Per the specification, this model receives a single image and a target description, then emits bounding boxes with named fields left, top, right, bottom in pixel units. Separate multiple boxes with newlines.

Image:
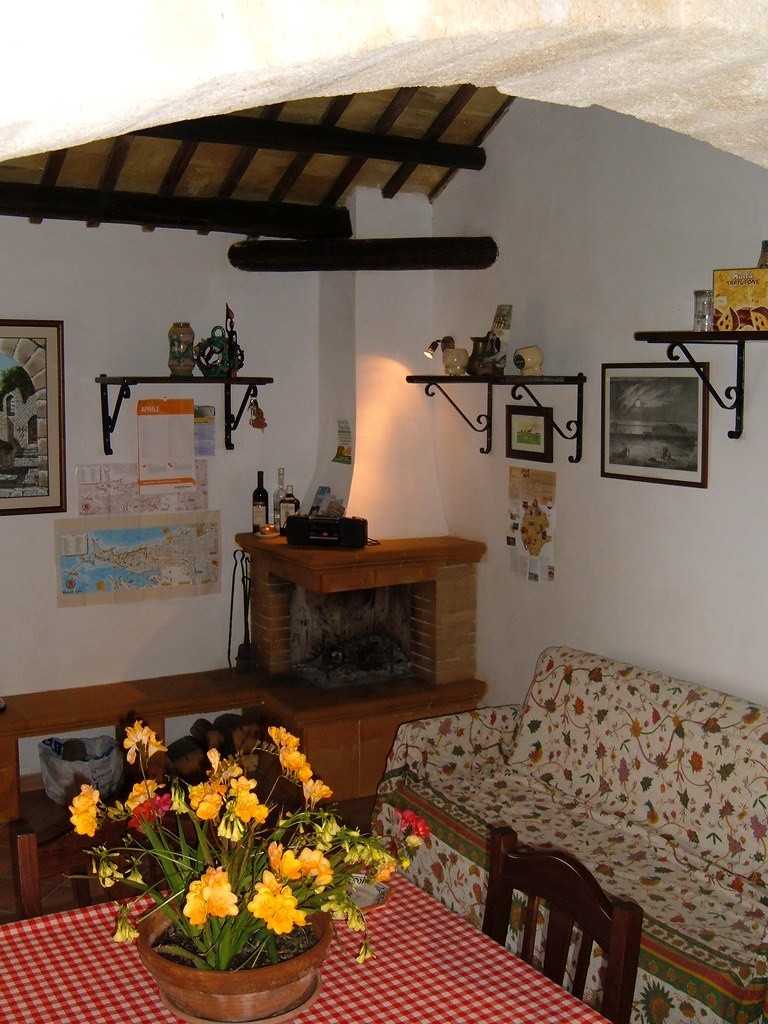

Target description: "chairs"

left=478, top=825, right=644, bottom=1024
left=8, top=801, right=223, bottom=918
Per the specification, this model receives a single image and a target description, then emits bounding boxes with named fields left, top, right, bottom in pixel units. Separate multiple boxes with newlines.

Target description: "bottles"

left=273, top=468, right=287, bottom=532
left=467, top=336, right=487, bottom=376
left=279, top=485, right=300, bottom=536
left=252, top=470, right=268, bottom=532
left=168, top=321, right=195, bottom=373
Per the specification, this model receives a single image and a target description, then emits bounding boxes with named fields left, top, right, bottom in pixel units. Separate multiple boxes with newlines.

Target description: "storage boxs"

left=713, top=268, right=768, bottom=331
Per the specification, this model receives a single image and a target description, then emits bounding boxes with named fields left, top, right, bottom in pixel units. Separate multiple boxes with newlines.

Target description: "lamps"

left=424, top=336, right=455, bottom=359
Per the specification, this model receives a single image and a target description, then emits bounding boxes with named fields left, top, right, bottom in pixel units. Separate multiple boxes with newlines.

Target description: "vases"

left=134, top=900, right=333, bottom=1024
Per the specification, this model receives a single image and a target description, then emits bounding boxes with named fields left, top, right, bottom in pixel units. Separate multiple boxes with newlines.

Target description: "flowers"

left=64, top=716, right=430, bottom=970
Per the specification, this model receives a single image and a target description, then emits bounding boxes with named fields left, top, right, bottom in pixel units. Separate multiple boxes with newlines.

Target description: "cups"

left=693, top=290, right=714, bottom=332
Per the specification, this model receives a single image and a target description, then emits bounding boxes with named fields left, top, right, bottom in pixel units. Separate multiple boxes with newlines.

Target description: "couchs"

left=366, top=644, right=768, bottom=1024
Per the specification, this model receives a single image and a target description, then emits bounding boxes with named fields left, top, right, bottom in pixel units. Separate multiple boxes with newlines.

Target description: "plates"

left=255, top=532, right=280, bottom=538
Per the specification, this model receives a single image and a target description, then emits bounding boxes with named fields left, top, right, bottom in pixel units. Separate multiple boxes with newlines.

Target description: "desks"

left=0, top=869, right=615, bottom=1024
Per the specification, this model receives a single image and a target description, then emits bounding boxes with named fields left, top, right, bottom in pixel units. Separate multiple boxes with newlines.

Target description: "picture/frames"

left=599, top=362, right=711, bottom=489
left=504, top=403, right=553, bottom=463
left=0, top=318, right=67, bottom=517
